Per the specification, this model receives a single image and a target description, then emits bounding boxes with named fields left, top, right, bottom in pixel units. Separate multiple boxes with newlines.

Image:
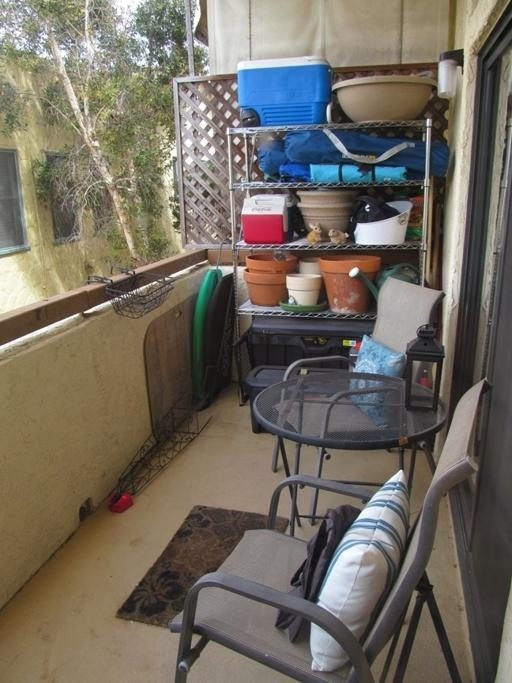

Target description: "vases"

left=244, top=191, right=381, bottom=315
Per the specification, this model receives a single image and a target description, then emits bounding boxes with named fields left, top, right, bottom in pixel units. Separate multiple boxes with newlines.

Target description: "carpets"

left=115, top=505, right=289, bottom=628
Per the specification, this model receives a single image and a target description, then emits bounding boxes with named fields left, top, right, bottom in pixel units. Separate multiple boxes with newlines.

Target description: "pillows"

left=309, top=469, right=410, bottom=672
left=349, top=335, right=405, bottom=426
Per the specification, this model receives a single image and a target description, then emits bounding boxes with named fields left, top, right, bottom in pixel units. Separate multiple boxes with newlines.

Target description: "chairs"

left=169, top=378, right=494, bottom=683
left=272, top=276, right=446, bottom=472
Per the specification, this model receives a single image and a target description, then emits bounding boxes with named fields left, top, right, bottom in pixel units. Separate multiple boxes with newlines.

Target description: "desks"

left=252, top=372, right=450, bottom=536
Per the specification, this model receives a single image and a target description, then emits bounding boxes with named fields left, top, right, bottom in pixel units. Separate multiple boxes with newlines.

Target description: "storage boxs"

left=237, top=56, right=332, bottom=127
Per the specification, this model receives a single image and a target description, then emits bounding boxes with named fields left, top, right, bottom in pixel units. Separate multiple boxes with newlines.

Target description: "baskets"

left=104, top=271, right=176, bottom=319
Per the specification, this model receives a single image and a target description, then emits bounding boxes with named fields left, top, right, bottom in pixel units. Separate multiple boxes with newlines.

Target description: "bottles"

left=437, top=52, right=457, bottom=99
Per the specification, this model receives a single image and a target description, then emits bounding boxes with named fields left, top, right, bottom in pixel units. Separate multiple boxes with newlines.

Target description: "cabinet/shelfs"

left=226, top=118, right=432, bottom=405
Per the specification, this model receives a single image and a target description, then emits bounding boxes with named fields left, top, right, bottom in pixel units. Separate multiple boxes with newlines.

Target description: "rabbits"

left=307, top=224, right=348, bottom=242
